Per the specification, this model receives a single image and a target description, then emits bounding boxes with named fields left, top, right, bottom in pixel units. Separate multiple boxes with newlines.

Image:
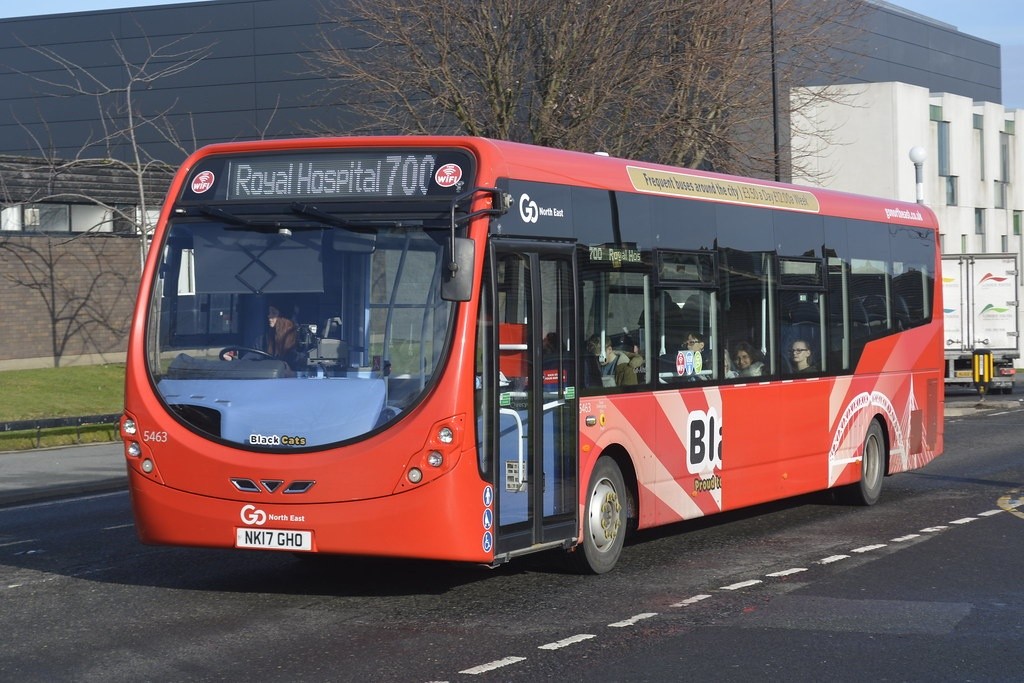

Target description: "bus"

left=119, top=136, right=944, bottom=574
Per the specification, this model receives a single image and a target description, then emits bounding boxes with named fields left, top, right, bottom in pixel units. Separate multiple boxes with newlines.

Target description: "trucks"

left=935, top=252, right=1020, bottom=395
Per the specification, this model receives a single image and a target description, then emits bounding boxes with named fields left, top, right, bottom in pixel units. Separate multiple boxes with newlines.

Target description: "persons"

left=542, top=292, right=817, bottom=389
left=222, top=305, right=307, bottom=372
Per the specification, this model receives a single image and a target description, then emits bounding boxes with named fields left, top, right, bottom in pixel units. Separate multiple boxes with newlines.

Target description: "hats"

left=264, top=296, right=296, bottom=315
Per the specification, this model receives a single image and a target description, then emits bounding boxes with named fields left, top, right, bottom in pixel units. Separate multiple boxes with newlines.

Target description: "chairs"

left=546, top=289, right=909, bottom=390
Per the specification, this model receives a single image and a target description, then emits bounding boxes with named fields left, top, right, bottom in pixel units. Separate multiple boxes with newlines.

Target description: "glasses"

left=790, top=348, right=808, bottom=354
left=682, top=339, right=702, bottom=348
